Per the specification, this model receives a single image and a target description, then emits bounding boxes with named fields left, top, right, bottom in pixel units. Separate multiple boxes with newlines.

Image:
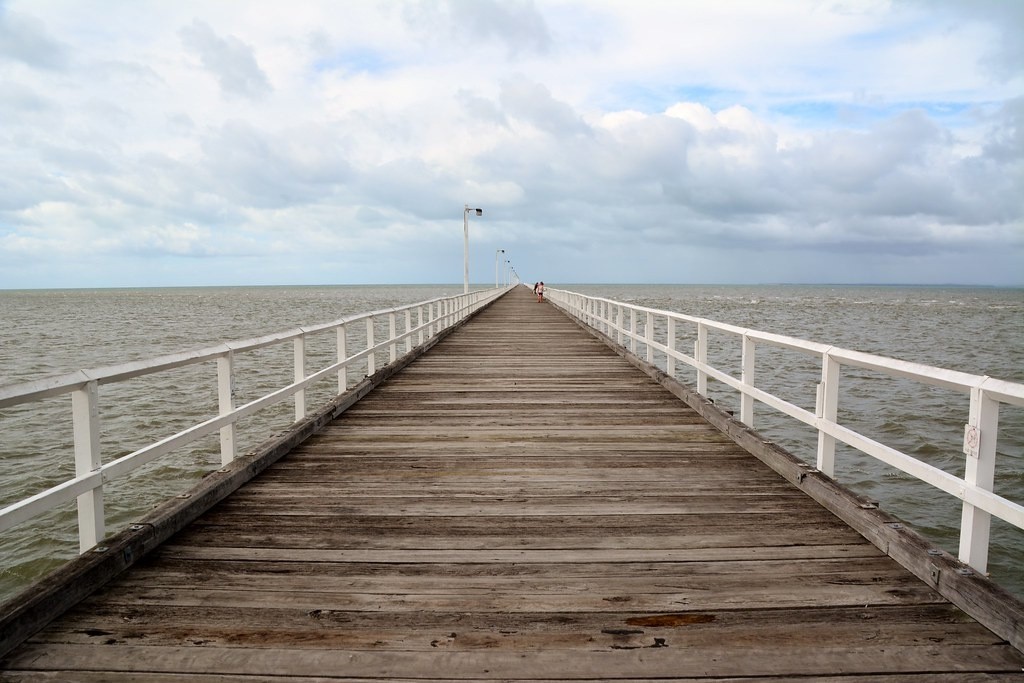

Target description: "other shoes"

left=541, top=301, right=544, bottom=303
left=538, top=301, right=540, bottom=303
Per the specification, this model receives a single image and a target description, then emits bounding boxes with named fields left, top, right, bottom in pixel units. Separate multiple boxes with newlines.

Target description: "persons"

left=535, top=281, right=544, bottom=303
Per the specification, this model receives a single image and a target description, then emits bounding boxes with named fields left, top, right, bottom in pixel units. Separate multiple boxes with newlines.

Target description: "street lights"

left=463, top=207, right=483, bottom=294
left=504, top=261, right=520, bottom=287
left=495, top=249, right=505, bottom=288
